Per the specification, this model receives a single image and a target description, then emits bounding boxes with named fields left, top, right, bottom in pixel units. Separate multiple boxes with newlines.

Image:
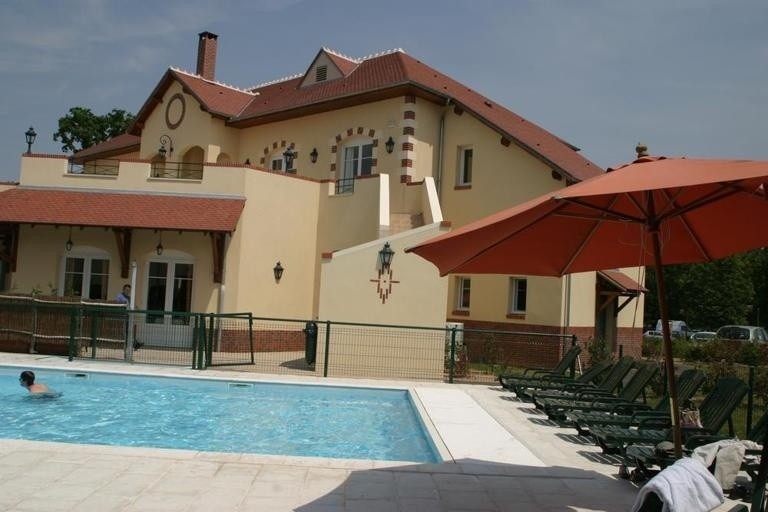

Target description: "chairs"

left=499, top=344, right=768, bottom=512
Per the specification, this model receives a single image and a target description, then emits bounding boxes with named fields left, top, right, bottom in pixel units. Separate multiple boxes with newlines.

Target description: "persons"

left=117, top=285, right=144, bottom=351
left=19, top=371, right=58, bottom=398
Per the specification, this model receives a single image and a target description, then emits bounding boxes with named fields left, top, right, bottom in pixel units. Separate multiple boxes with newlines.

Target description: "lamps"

left=385, top=137, right=395, bottom=153
left=156, top=229, right=163, bottom=255
left=274, top=261, right=284, bottom=279
left=158, top=135, right=173, bottom=160
left=378, top=242, right=396, bottom=265
left=283, top=146, right=296, bottom=170
left=66, top=226, right=73, bottom=250
left=244, top=159, right=251, bottom=165
left=25, top=125, right=37, bottom=152
left=310, top=148, right=318, bottom=163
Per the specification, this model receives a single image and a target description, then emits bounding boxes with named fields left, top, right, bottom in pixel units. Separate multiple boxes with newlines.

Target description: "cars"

left=643, top=319, right=768, bottom=344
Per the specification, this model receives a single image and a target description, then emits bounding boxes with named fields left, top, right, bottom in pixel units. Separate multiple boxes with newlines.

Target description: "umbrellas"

left=403, top=140, right=765, bottom=458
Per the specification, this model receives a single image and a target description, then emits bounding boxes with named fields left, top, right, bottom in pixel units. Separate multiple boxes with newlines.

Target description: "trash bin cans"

left=305, top=321, right=318, bottom=365
